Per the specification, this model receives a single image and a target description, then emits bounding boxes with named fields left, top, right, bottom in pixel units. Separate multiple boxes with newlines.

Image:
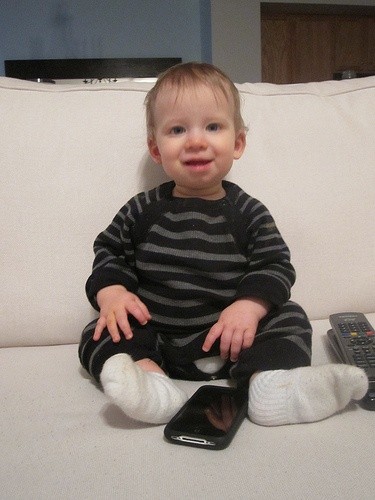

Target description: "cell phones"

left=163, top=385, right=248, bottom=450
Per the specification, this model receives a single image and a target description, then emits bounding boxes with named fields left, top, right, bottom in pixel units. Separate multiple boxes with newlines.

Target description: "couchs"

left=0, top=75, right=375, bottom=500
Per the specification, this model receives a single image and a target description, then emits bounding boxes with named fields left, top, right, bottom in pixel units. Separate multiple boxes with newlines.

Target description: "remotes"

left=327, top=329, right=375, bottom=411
left=330, top=311, right=375, bottom=389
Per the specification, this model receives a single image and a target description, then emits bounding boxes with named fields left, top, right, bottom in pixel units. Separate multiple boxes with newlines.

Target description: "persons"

left=206, top=393, right=238, bottom=432
left=77, top=61, right=367, bottom=427
